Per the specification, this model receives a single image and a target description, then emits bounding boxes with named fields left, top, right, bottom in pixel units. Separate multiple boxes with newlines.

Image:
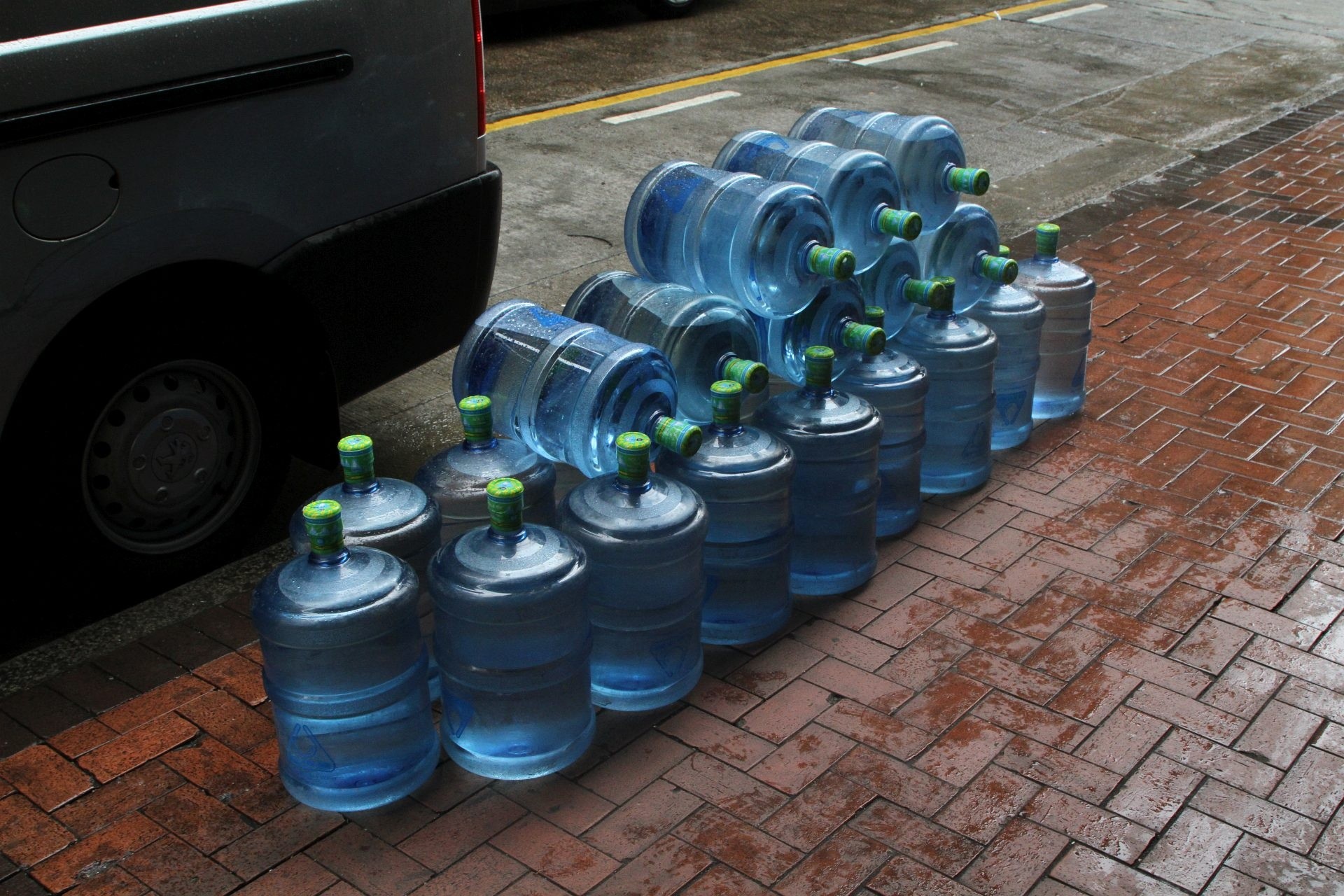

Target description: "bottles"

left=408, top=394, right=556, bottom=572
left=250, top=500, right=442, bottom=811
left=1004, top=222, right=1096, bottom=418
left=287, top=433, right=446, bottom=705
left=451, top=104, right=1047, bottom=712
left=424, top=476, right=599, bottom=782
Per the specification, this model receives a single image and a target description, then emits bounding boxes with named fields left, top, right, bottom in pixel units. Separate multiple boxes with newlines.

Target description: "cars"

left=1, top=1, right=508, bottom=611
